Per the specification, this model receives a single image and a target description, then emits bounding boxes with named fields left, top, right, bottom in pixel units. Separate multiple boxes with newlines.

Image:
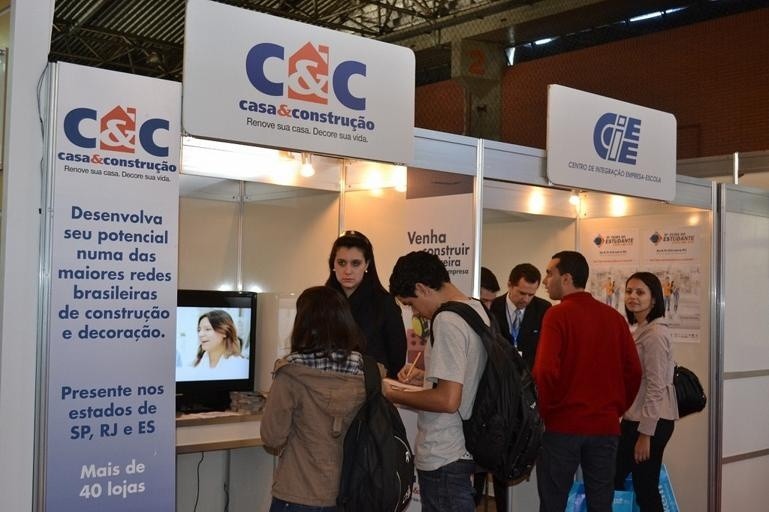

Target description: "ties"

left=511, top=309, right=521, bottom=346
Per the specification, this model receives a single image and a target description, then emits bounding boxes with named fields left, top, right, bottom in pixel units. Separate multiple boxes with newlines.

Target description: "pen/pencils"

left=406, top=351, right=422, bottom=377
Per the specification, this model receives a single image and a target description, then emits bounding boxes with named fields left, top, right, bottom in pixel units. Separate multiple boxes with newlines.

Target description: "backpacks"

left=430, top=296, right=543, bottom=483
left=336, top=353, right=415, bottom=512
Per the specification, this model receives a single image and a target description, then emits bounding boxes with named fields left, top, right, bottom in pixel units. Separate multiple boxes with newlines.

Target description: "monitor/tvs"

left=176, top=289, right=257, bottom=414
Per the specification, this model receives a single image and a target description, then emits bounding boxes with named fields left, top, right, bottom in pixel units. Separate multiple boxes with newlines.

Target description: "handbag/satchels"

left=673, top=365, right=707, bottom=418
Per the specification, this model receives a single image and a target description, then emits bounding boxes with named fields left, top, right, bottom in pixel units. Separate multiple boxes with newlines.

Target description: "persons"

left=531, top=250, right=644, bottom=512
left=602, top=277, right=620, bottom=312
left=661, top=277, right=680, bottom=311
left=382, top=250, right=497, bottom=511
left=615, top=272, right=680, bottom=512
left=185, top=307, right=251, bottom=380
left=325, top=230, right=408, bottom=379
left=481, top=264, right=499, bottom=311
left=260, top=285, right=382, bottom=511
left=493, top=263, right=551, bottom=368
